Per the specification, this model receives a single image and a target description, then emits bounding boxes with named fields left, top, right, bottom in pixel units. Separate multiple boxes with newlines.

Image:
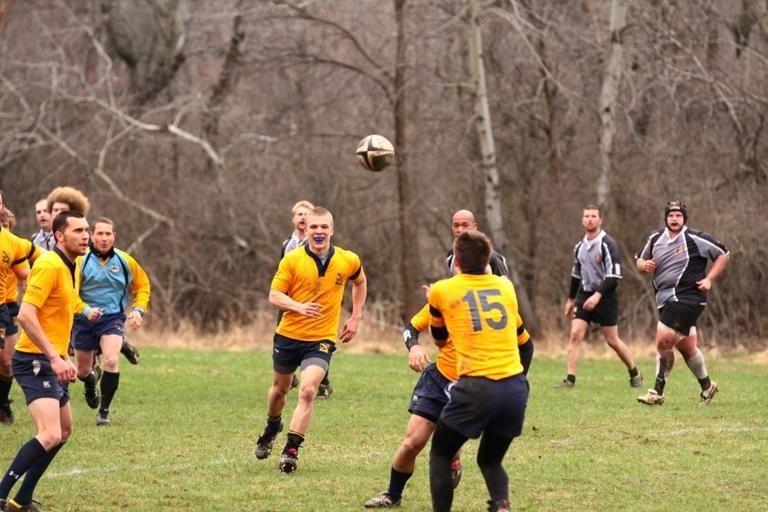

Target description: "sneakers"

left=254, top=376, right=334, bottom=460
left=120, top=336, right=141, bottom=367
left=362, top=451, right=513, bottom=512
left=94, top=406, right=112, bottom=427
left=551, top=377, right=577, bottom=389
left=629, top=371, right=646, bottom=389
left=694, top=381, right=720, bottom=407
left=637, top=387, right=666, bottom=407
left=278, top=446, right=299, bottom=472
left=82, top=370, right=101, bottom=409
left=0, top=497, right=42, bottom=512
left=0, top=395, right=16, bottom=426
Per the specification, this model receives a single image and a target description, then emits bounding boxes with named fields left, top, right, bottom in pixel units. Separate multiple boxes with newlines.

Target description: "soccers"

left=357, top=136, right=394, bottom=171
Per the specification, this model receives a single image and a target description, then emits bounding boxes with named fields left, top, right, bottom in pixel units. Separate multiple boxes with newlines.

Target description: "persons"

left=276, top=200, right=333, bottom=398
left=0, top=187, right=150, bottom=429
left=559, top=202, right=643, bottom=389
left=253, top=207, right=367, bottom=474
left=363, top=285, right=536, bottom=505
left=447, top=209, right=510, bottom=280
left=427, top=231, right=529, bottom=510
left=634, top=200, right=731, bottom=404
left=1, top=209, right=89, bottom=511
